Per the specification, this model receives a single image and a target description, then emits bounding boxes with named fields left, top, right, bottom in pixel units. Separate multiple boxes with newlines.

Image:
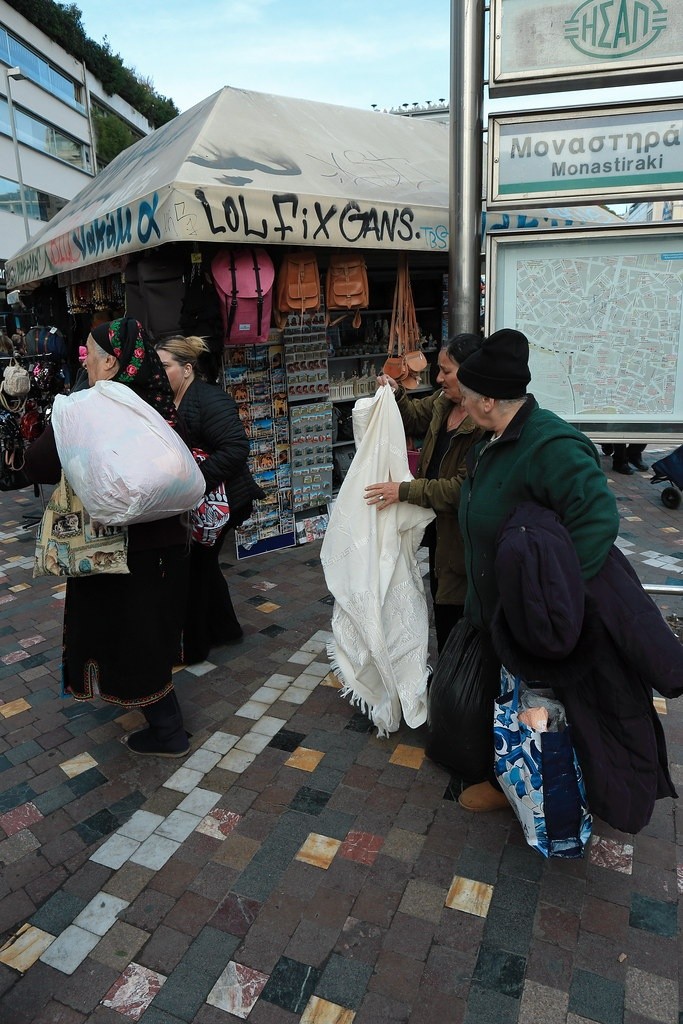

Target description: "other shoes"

left=602, top=443, right=614, bottom=456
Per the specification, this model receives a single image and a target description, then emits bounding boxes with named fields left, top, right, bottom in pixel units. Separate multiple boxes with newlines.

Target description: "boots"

left=125, top=687, right=191, bottom=758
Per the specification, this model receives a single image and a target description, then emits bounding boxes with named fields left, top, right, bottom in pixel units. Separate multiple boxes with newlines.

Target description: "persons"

left=280, top=450, right=288, bottom=465
left=154, top=335, right=265, bottom=666
left=25, top=317, right=190, bottom=756
left=611, top=443, right=650, bottom=474
left=0, top=329, right=43, bottom=489
left=457, top=329, right=619, bottom=812
left=365, top=333, right=488, bottom=731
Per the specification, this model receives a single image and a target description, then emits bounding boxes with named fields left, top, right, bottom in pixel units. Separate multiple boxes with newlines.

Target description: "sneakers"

left=457, top=780, right=511, bottom=812
left=626, top=447, right=650, bottom=470
left=612, top=464, right=634, bottom=475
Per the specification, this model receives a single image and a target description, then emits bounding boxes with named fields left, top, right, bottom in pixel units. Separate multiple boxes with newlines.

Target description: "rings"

left=380, top=495, right=384, bottom=501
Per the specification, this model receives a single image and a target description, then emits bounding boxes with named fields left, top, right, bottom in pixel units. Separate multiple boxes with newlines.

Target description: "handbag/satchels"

left=52, top=379, right=208, bottom=526
left=0, top=281, right=78, bottom=498
left=383, top=350, right=427, bottom=389
left=181, top=447, right=230, bottom=547
left=493, top=664, right=592, bottom=860
left=32, top=468, right=130, bottom=579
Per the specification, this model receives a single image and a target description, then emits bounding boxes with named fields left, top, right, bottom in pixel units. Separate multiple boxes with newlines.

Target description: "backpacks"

left=124, top=244, right=370, bottom=386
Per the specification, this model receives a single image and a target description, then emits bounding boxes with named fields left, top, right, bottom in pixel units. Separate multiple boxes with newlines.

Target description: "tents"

left=4, top=86, right=627, bottom=289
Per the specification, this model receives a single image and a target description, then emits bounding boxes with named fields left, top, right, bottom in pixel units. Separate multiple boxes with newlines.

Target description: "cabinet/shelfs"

left=222, top=304, right=431, bottom=560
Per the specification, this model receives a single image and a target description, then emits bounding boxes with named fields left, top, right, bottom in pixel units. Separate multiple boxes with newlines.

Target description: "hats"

left=456, top=328, right=531, bottom=399
